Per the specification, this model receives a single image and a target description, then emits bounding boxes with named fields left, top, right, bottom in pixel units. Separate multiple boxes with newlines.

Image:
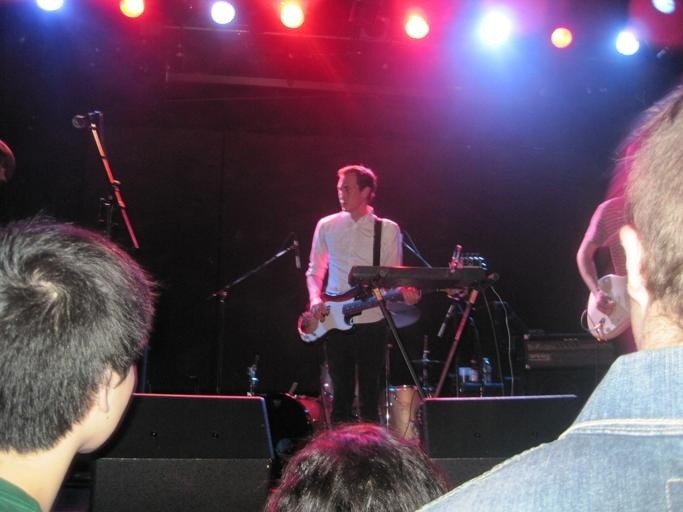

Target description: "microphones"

left=73, top=110, right=103, bottom=128
left=293, top=237, right=302, bottom=270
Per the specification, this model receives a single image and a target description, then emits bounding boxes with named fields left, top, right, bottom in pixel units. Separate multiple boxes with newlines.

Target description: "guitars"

left=296, top=286, right=467, bottom=343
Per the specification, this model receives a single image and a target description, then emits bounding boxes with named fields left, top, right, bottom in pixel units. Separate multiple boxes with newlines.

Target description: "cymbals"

left=402, top=354, right=451, bottom=368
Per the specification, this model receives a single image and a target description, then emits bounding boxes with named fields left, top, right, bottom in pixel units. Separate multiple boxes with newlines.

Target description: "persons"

left=415, top=90, right=683, bottom=512
left=0, top=218, right=153, bottom=511
left=265, top=421, right=447, bottom=511
left=575, top=193, right=638, bottom=354
left=304, top=164, right=424, bottom=429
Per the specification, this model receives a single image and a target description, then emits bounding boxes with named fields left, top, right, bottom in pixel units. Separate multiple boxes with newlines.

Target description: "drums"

left=250, top=392, right=325, bottom=459
left=379, top=385, right=435, bottom=447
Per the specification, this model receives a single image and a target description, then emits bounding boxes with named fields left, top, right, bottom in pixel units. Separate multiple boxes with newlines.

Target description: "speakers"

left=90, top=393, right=276, bottom=511
left=419, top=395, right=581, bottom=501
